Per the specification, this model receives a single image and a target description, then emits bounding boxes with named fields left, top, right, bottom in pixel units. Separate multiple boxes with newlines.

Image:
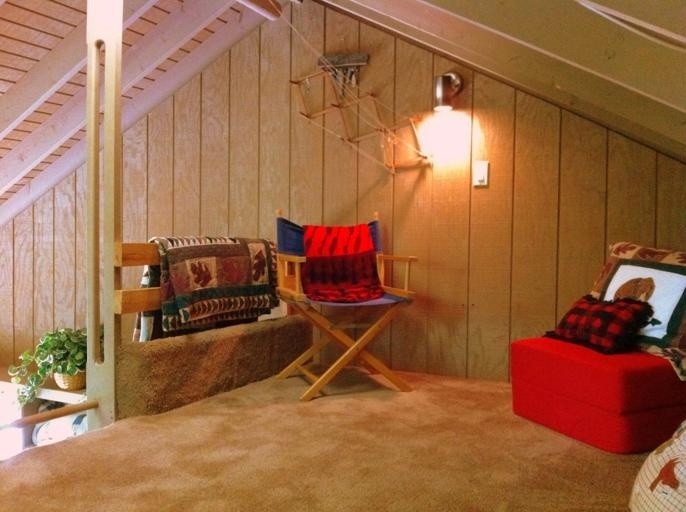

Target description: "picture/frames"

left=550, top=297, right=651, bottom=353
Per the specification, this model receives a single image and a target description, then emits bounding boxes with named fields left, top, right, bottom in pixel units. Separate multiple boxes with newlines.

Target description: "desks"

left=15, top=373, right=87, bottom=450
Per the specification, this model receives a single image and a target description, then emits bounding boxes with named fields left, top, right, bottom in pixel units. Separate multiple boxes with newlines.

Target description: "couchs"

left=512, top=242, right=686, bottom=453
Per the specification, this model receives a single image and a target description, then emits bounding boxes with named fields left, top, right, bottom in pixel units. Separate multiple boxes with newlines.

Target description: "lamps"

left=431, top=72, right=461, bottom=113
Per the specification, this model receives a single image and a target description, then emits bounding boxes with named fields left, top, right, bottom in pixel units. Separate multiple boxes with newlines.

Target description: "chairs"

left=273, top=210, right=416, bottom=401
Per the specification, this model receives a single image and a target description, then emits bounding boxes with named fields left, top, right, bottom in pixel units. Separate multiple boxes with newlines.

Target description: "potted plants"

left=10, top=326, right=104, bottom=399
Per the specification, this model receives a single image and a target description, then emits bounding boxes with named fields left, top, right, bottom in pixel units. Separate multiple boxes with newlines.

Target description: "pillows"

left=589, top=259, right=686, bottom=352
